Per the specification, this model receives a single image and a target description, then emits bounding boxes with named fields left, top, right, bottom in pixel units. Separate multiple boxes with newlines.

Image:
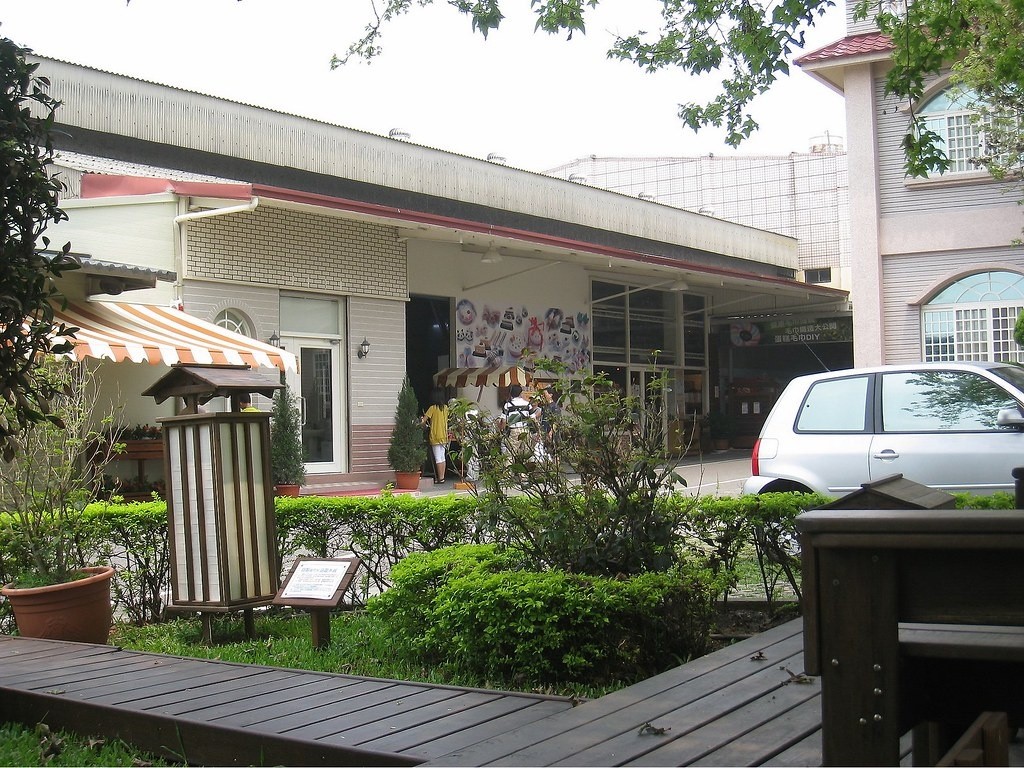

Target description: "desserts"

left=455, top=303, right=528, bottom=368
left=545, top=309, right=591, bottom=374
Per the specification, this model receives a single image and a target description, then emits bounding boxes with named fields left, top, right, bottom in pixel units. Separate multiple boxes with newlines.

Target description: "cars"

left=742, top=362, right=1024, bottom=565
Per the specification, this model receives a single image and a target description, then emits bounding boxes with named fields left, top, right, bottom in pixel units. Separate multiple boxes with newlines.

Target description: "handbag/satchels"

left=527, top=437, right=553, bottom=463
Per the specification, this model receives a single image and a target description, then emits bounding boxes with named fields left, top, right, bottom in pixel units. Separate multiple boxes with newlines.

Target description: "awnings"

left=0, top=301, right=302, bottom=375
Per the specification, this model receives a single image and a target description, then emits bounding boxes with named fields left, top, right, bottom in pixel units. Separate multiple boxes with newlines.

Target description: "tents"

left=432, top=366, right=530, bottom=403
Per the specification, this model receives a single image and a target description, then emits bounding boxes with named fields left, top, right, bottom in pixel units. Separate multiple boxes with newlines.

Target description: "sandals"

left=435, top=478, right=444, bottom=483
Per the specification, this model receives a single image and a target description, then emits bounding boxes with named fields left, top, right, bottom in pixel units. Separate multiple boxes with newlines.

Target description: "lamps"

left=480, top=239, right=503, bottom=264
left=268, top=330, right=280, bottom=347
left=358, top=336, right=371, bottom=359
left=669, top=272, right=689, bottom=291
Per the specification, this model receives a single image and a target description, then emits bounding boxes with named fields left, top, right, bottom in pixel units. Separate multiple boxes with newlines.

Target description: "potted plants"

left=270, top=381, right=308, bottom=498
left=0, top=342, right=131, bottom=644
left=701, top=408, right=736, bottom=450
left=386, top=370, right=429, bottom=491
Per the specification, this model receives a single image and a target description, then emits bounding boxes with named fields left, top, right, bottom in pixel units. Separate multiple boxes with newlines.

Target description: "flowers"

left=94, top=423, right=162, bottom=441
left=103, top=471, right=166, bottom=494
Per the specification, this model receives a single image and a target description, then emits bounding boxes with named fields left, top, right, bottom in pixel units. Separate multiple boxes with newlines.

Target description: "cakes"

left=529, top=325, right=544, bottom=346
left=507, top=335, right=524, bottom=356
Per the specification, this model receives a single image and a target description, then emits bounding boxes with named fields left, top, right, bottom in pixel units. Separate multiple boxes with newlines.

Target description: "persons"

left=417, top=385, right=562, bottom=491
left=176, top=393, right=262, bottom=415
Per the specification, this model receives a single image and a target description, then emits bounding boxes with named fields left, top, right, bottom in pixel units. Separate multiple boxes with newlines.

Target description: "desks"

left=86, top=439, right=163, bottom=496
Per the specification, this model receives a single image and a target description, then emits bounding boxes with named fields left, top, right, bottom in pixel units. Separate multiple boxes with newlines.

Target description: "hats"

left=529, top=395, right=540, bottom=400
